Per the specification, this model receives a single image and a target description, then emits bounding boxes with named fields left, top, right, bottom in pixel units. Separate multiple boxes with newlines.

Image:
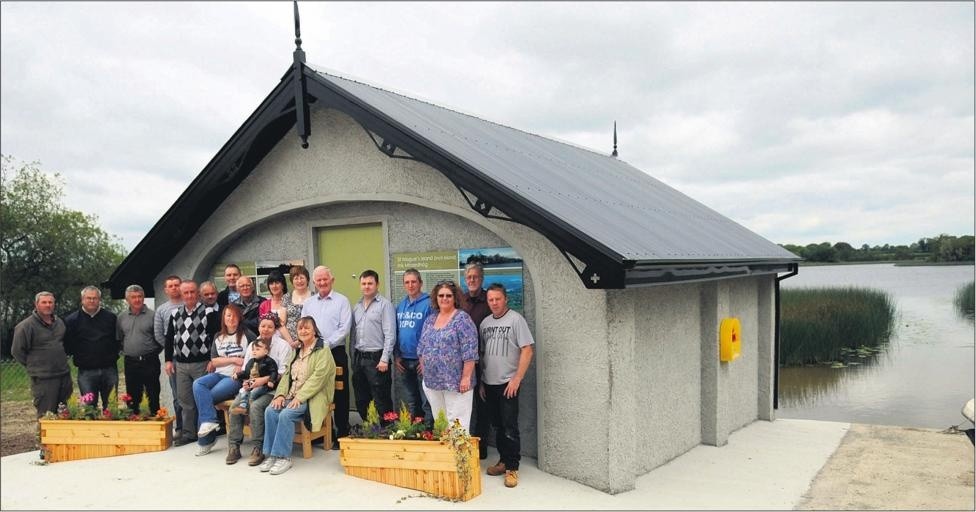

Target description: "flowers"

left=362, top=402, right=449, bottom=441
left=58, top=391, right=168, bottom=421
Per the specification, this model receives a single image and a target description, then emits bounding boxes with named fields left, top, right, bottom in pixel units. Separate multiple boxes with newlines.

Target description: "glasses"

left=437, top=280, right=454, bottom=286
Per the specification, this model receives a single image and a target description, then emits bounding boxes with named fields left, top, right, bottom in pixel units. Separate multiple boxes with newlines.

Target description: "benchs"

left=214, top=366, right=344, bottom=459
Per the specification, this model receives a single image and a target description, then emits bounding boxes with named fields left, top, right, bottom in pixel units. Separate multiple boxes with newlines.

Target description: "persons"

left=9, top=262, right=534, bottom=490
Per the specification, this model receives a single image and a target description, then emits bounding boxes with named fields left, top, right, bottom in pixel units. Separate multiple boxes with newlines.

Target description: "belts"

left=125, top=354, right=159, bottom=361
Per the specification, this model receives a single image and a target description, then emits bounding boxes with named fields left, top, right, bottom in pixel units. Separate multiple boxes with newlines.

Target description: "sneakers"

left=226, top=446, right=242, bottom=465
left=487, top=462, right=506, bottom=476
left=246, top=446, right=264, bottom=466
left=193, top=438, right=219, bottom=457
left=269, top=455, right=293, bottom=475
left=172, top=430, right=198, bottom=446
left=476, top=440, right=488, bottom=460
left=504, top=469, right=519, bottom=488
left=195, top=423, right=221, bottom=437
left=332, top=433, right=348, bottom=451
left=312, top=437, right=324, bottom=446
left=230, top=406, right=249, bottom=416
left=259, top=456, right=278, bottom=472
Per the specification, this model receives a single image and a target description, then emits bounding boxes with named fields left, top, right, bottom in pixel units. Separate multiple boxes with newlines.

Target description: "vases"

left=40, top=418, right=176, bottom=462
left=338, top=437, right=482, bottom=500
